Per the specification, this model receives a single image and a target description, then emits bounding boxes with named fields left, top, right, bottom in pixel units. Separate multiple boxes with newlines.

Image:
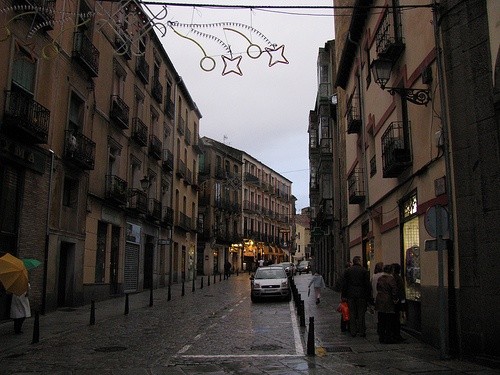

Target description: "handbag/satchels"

left=308, top=287, right=310, bottom=297
left=365, top=305, right=375, bottom=321
left=336, top=310, right=342, bottom=322
left=399, top=311, right=407, bottom=325
left=363, top=282, right=374, bottom=305
left=336, top=291, right=342, bottom=303
left=375, top=293, right=382, bottom=310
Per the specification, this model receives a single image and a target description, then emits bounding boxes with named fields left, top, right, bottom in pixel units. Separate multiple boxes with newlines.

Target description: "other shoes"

left=16, top=331, right=24, bottom=334
left=352, top=335, right=356, bottom=337
left=360, top=335, right=366, bottom=337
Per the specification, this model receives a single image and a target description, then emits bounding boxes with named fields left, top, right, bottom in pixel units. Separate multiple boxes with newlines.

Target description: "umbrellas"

left=0, top=253, right=28, bottom=296
left=22, top=259, right=41, bottom=271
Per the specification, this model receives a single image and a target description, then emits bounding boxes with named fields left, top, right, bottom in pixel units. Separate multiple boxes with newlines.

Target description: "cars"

left=249, top=266, right=291, bottom=302
left=268, top=262, right=296, bottom=281
left=297, top=261, right=311, bottom=274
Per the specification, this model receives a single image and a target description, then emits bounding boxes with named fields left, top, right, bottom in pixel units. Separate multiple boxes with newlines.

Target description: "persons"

left=10, top=283, right=31, bottom=334
left=374, top=264, right=400, bottom=344
left=247, top=258, right=274, bottom=271
left=337, top=297, right=350, bottom=335
left=371, top=262, right=385, bottom=326
left=391, top=263, right=405, bottom=343
left=308, top=270, right=326, bottom=306
left=224, top=259, right=232, bottom=277
left=341, top=256, right=370, bottom=337
